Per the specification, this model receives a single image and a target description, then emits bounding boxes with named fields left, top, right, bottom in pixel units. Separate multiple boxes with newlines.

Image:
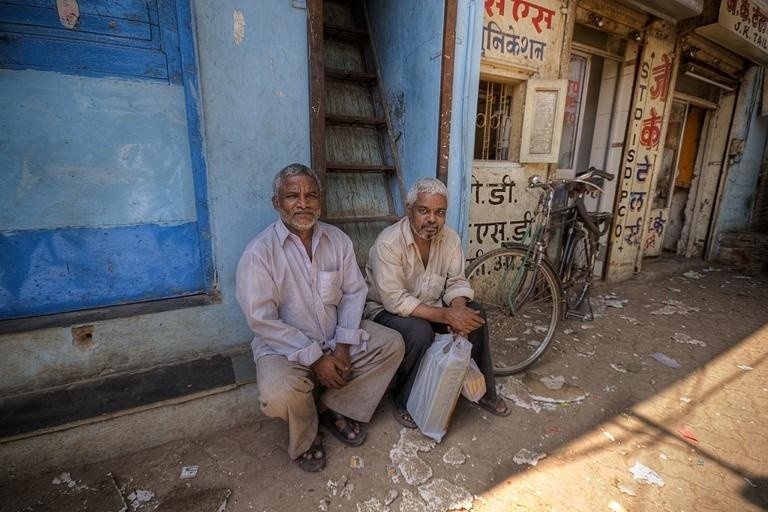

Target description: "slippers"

left=319, top=404, right=367, bottom=447
left=389, top=392, right=418, bottom=430
left=296, top=434, right=326, bottom=472
left=479, top=393, right=512, bottom=418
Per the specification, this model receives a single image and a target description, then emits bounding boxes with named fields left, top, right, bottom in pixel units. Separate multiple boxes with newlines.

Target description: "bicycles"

left=461, top=167, right=615, bottom=376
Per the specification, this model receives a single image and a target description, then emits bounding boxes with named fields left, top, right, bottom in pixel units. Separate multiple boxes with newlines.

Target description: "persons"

left=233, top=162, right=406, bottom=473
left=363, top=176, right=510, bottom=429
